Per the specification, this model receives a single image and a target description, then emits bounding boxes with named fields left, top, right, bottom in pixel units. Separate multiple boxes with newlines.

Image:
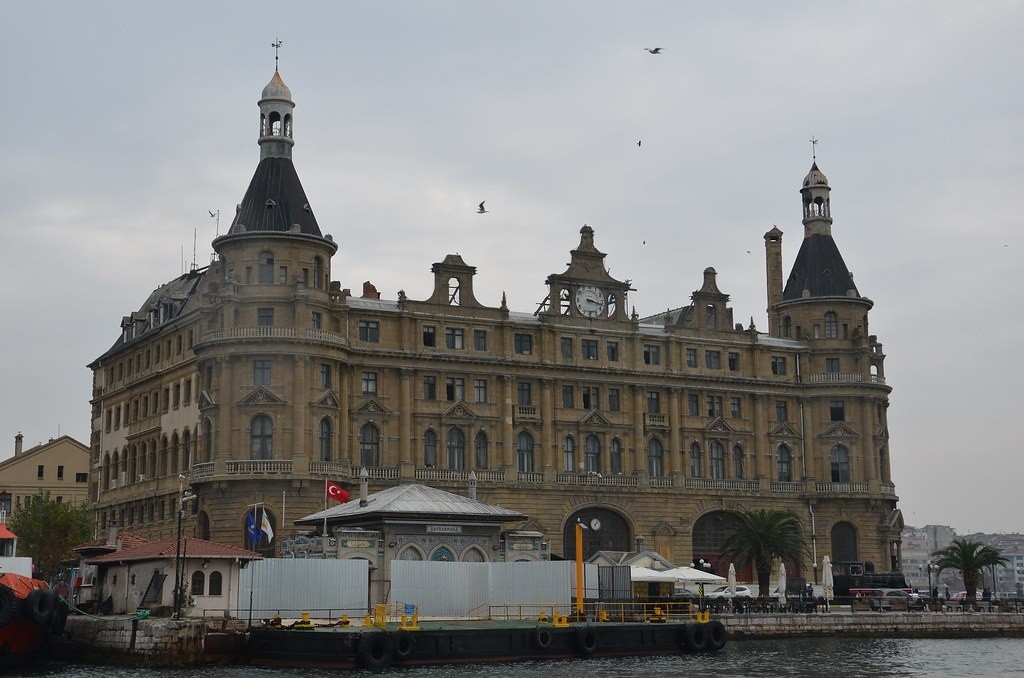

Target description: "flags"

left=246, top=511, right=261, bottom=545
left=327, top=481, right=350, bottom=503
left=261, top=508, right=274, bottom=543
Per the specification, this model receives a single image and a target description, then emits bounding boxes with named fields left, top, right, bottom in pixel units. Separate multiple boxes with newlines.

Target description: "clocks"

left=575, top=285, right=605, bottom=318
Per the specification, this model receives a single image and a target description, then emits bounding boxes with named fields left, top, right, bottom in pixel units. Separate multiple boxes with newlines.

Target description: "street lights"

left=919, top=560, right=939, bottom=597
left=690, top=559, right=712, bottom=597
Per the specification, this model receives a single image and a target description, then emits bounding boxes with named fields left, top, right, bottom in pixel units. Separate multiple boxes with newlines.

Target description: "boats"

left=0, top=510, right=69, bottom=656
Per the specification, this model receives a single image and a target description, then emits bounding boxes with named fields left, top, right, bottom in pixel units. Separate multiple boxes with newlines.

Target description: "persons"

left=982, top=588, right=991, bottom=601
left=945, top=587, right=950, bottom=599
left=633, top=592, right=644, bottom=616
left=933, top=586, right=938, bottom=597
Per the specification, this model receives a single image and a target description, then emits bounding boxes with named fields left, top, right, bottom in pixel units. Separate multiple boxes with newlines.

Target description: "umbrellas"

left=822, top=554, right=835, bottom=613
left=778, top=564, right=786, bottom=606
left=728, top=562, right=737, bottom=612
left=631, top=566, right=727, bottom=610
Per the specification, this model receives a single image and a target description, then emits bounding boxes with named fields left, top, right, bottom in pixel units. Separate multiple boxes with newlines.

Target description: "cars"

left=867, top=589, right=915, bottom=610
left=706, top=586, right=753, bottom=598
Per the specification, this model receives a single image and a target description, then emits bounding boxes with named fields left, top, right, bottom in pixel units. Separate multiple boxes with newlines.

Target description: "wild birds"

left=208, top=209, right=217, bottom=218
left=476, top=201, right=490, bottom=215
left=746, top=250, right=751, bottom=253
left=636, top=140, right=642, bottom=148
left=643, top=47, right=664, bottom=56
left=641, top=241, right=648, bottom=245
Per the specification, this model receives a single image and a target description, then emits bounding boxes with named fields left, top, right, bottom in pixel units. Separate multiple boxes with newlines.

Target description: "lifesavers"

left=33, top=594, right=49, bottom=624
left=0, top=583, right=17, bottom=628
left=684, top=623, right=709, bottom=653
left=392, top=630, right=416, bottom=661
left=358, top=632, right=394, bottom=671
left=705, top=621, right=728, bottom=651
left=578, top=628, right=599, bottom=654
left=52, top=603, right=68, bottom=636
left=533, top=629, right=551, bottom=650
